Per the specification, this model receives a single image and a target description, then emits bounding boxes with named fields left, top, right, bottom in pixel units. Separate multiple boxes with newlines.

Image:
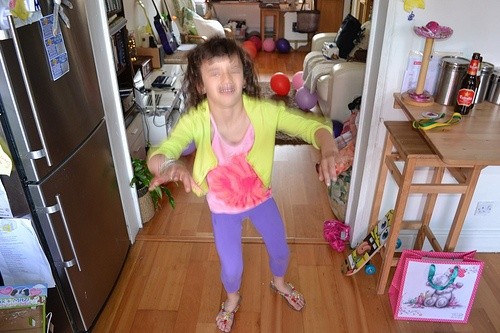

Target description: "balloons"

left=270, top=71, right=317, bottom=110
left=242, top=36, right=291, bottom=60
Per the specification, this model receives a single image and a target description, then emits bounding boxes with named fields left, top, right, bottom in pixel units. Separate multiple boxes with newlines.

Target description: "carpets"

left=258, top=81, right=322, bottom=146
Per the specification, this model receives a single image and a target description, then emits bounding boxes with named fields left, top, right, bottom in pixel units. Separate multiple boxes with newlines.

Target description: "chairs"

left=188, top=26, right=234, bottom=45
left=292, top=10, right=321, bottom=52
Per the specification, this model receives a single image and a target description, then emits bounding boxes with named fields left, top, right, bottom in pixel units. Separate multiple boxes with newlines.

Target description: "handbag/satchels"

left=387, top=249, right=486, bottom=324
left=296, top=0, right=321, bottom=34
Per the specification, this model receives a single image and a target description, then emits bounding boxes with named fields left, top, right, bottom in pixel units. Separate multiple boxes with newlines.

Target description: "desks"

left=259, top=8, right=299, bottom=43
left=134, top=63, right=191, bottom=147
left=393, top=92, right=500, bottom=167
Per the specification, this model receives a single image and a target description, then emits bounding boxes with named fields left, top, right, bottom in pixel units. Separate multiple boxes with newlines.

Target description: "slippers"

left=270, top=279, right=306, bottom=311
left=215, top=295, right=241, bottom=333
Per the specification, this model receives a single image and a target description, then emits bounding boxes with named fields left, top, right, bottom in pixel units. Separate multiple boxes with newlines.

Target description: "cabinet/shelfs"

left=105, top=0, right=141, bottom=130
left=356, top=0, right=372, bottom=25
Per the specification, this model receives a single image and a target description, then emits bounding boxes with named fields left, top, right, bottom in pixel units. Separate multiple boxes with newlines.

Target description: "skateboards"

left=342, top=208, right=401, bottom=276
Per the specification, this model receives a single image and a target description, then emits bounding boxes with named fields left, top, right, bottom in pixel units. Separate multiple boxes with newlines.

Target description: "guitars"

left=136, top=0, right=181, bottom=54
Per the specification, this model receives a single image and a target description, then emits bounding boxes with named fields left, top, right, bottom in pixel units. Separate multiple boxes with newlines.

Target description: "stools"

left=365, top=119, right=482, bottom=295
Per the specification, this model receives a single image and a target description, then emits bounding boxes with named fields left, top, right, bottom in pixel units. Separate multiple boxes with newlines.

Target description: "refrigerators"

left=0, top=9, right=131, bottom=333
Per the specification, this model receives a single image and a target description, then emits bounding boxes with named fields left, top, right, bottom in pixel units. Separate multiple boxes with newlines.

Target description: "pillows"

left=183, top=7, right=204, bottom=34
left=193, top=16, right=227, bottom=45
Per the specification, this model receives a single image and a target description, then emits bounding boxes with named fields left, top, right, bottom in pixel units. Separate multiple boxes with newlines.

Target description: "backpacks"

left=334, top=13, right=367, bottom=59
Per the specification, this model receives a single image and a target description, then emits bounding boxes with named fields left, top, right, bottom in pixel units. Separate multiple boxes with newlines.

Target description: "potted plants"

left=128, top=155, right=176, bottom=224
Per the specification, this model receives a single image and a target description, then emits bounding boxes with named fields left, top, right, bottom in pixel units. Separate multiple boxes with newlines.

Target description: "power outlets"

left=473, top=200, right=494, bottom=216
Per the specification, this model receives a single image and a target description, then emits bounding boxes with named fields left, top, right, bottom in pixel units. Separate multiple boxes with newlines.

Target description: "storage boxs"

left=135, top=44, right=164, bottom=70
left=132, top=56, right=155, bottom=81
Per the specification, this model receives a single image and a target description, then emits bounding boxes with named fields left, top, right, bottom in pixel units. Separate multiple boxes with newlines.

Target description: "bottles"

left=470, top=56, right=485, bottom=109
left=453, top=52, right=480, bottom=115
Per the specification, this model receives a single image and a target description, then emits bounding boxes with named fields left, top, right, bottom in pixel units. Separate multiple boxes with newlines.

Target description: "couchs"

left=310, top=20, right=371, bottom=123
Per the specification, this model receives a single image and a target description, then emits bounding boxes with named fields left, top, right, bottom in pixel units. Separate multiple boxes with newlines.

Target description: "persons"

left=147, top=36, right=340, bottom=332
left=317, top=111, right=358, bottom=177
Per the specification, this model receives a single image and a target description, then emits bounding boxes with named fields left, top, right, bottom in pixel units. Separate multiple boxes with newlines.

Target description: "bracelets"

left=159, top=159, right=175, bottom=173
left=350, top=124, right=355, bottom=126
